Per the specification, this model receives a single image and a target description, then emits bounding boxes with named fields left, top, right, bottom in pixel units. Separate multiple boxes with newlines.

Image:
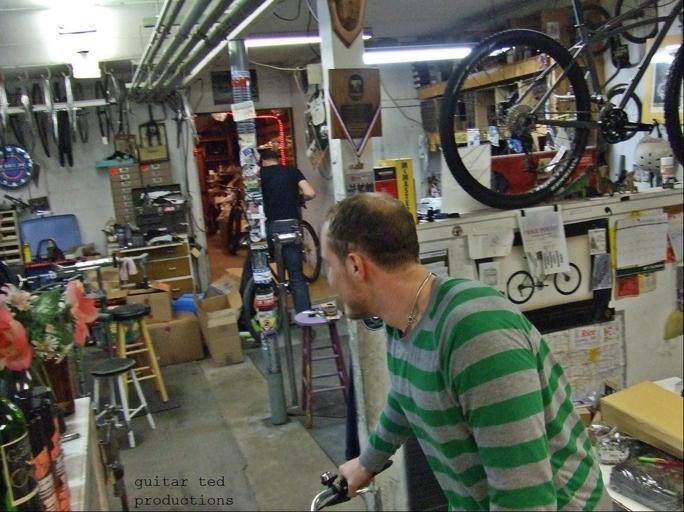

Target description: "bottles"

left=0, top=346, right=76, bottom=511
left=23, top=243, right=32, bottom=265
left=660, top=157, right=675, bottom=184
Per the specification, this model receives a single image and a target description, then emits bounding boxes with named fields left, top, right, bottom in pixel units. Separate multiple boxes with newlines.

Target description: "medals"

left=350, top=162, right=364, bottom=170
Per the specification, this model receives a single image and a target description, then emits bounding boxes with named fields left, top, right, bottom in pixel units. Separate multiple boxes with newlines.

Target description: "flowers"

left=1, top=275, right=100, bottom=375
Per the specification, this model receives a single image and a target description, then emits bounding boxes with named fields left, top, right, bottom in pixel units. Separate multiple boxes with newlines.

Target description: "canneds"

left=22, top=243, right=32, bottom=263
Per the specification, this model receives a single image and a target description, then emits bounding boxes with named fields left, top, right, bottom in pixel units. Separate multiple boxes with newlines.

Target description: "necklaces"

left=402, top=272, right=432, bottom=336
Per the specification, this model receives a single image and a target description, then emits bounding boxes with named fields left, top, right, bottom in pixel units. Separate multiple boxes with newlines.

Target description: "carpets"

left=114, top=384, right=180, bottom=422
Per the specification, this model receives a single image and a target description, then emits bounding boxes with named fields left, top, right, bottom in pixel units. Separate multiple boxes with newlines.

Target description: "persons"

left=319, top=190, right=616, bottom=512
left=238, top=150, right=316, bottom=332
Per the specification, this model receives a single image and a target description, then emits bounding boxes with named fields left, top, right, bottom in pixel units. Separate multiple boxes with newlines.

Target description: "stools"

left=89, top=301, right=169, bottom=448
left=294, top=307, right=350, bottom=429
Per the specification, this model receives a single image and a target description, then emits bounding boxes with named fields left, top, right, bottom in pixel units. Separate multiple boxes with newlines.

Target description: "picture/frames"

left=639, top=34, right=684, bottom=126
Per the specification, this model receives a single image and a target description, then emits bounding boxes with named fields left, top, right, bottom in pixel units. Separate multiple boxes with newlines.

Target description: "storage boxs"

left=91, top=267, right=256, bottom=369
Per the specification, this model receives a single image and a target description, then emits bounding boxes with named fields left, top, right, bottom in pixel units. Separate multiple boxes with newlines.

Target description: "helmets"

left=633, top=135, right=678, bottom=177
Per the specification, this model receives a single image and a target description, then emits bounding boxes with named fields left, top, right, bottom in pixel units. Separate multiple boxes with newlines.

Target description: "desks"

left=60, top=397, right=109, bottom=511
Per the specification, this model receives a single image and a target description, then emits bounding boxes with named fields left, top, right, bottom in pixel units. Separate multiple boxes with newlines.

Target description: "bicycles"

left=242, top=203, right=321, bottom=345
left=438, top=1, right=683, bottom=211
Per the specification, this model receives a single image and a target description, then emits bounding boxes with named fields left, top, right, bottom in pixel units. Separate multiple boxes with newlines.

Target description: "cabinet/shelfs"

left=118, top=237, right=200, bottom=301
left=415, top=54, right=607, bottom=147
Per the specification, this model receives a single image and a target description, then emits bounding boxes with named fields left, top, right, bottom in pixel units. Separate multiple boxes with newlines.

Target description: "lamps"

left=240, top=27, right=480, bottom=65
